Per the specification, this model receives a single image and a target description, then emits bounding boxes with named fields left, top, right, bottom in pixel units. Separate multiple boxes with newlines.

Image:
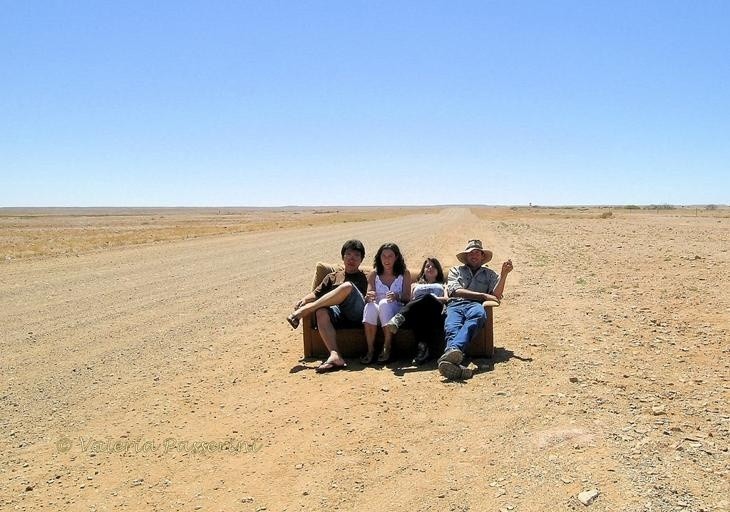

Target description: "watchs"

left=397, top=292, right=401, bottom=302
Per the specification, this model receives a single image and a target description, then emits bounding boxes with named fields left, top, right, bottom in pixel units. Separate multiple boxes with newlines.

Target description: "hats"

left=456, top=239, right=493, bottom=266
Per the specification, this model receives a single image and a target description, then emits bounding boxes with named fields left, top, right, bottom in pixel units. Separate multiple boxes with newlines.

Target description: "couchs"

left=303, top=262, right=493, bottom=361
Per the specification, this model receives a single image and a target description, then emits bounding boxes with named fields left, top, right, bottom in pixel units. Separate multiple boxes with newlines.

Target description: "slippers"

left=316, top=361, right=347, bottom=372
left=286, top=313, right=299, bottom=329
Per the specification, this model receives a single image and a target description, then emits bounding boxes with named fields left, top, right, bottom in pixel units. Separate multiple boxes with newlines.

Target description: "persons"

left=287, top=240, right=370, bottom=370
left=409, top=258, right=449, bottom=364
left=437, top=239, right=514, bottom=380
left=361, top=243, right=410, bottom=363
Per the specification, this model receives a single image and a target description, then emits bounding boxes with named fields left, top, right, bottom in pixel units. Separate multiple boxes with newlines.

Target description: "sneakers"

left=437, top=348, right=472, bottom=379
left=411, top=342, right=429, bottom=365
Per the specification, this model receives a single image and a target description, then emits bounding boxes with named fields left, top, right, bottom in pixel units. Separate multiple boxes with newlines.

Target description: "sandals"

left=376, top=345, right=391, bottom=362
left=360, top=352, right=373, bottom=364
